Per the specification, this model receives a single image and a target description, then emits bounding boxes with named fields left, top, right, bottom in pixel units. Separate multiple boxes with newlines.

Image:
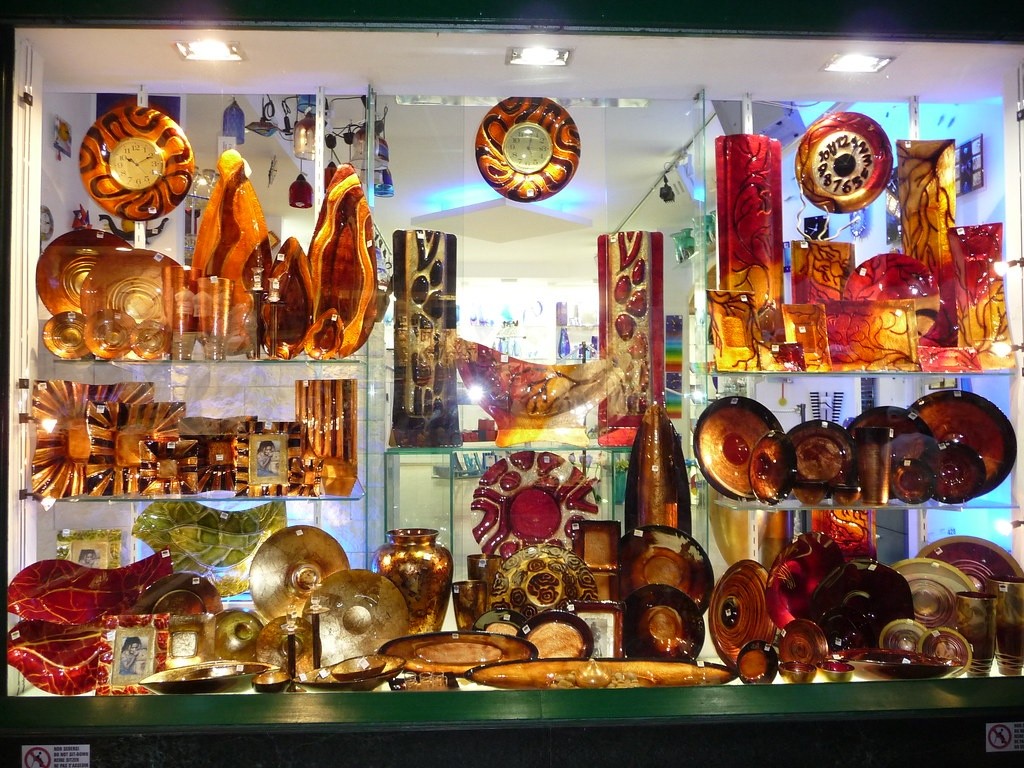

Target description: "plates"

left=120, top=452, right=738, bottom=692
left=887, top=558, right=980, bottom=630
left=844, top=253, right=940, bottom=339
left=618, top=524, right=845, bottom=684
left=694, top=390, right=1017, bottom=507
left=879, top=619, right=929, bottom=654
left=37, top=229, right=185, bottom=361
left=917, top=536, right=1024, bottom=593
left=848, top=661, right=963, bottom=679
left=810, top=561, right=915, bottom=655
left=917, top=627, right=972, bottom=677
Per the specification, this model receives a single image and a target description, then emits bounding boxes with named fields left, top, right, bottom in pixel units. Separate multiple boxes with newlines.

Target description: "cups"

left=986, top=576, right=1024, bottom=676
left=161, top=266, right=233, bottom=361
left=954, top=591, right=998, bottom=673
left=854, top=426, right=894, bottom=506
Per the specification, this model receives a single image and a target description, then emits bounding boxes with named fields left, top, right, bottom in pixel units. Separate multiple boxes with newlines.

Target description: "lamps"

left=659, top=170, right=675, bottom=205
left=244, top=94, right=394, bottom=208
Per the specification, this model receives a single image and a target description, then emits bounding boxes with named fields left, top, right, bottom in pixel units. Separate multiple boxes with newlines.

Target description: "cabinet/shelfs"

left=0, top=357, right=1023, bottom=767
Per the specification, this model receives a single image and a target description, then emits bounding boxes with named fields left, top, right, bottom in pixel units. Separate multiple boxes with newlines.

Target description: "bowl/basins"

left=792, top=480, right=828, bottom=505
left=832, top=486, right=862, bottom=506
left=815, top=662, right=855, bottom=681
left=778, top=661, right=817, bottom=683
left=251, top=672, right=292, bottom=692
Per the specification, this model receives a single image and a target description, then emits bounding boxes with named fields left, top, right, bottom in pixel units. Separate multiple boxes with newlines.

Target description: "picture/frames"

left=235, top=419, right=303, bottom=498
left=558, top=596, right=623, bottom=658
left=96, top=613, right=170, bottom=696
left=56, top=528, right=121, bottom=570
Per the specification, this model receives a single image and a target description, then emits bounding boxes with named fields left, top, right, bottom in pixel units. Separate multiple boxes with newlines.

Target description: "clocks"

left=40, top=205, right=54, bottom=241
left=804, top=215, right=829, bottom=240
left=502, top=122, right=553, bottom=174
left=812, top=130, right=876, bottom=196
left=109, top=138, right=166, bottom=191
